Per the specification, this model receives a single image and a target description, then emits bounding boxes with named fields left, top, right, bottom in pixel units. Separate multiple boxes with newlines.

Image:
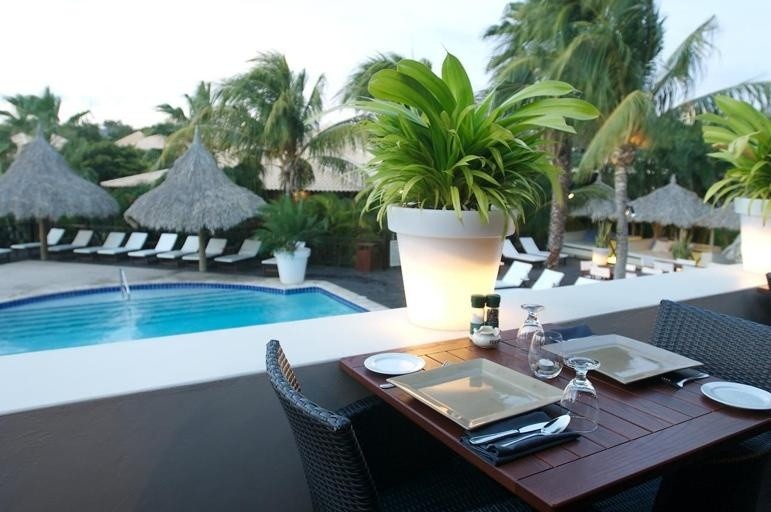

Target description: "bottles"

left=486, top=294, right=501, bottom=329
left=469, top=295, right=485, bottom=337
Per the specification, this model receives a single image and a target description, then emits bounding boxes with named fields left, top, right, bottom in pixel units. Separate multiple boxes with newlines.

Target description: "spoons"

left=500, top=415, right=571, bottom=450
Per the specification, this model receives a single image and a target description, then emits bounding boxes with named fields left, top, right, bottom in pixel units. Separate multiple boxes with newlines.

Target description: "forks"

left=660, top=372, right=710, bottom=389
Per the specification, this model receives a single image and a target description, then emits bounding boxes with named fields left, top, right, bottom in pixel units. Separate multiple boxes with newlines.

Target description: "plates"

left=386, top=357, right=569, bottom=432
left=700, top=381, right=771, bottom=410
left=364, top=352, right=426, bottom=376
left=540, top=334, right=705, bottom=385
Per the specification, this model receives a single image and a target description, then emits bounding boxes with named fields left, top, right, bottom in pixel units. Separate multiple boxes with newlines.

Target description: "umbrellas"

left=694, top=196, right=740, bottom=231
left=122, top=125, right=267, bottom=272
left=1, top=120, right=122, bottom=261
left=622, top=174, right=713, bottom=243
left=563, top=171, right=631, bottom=221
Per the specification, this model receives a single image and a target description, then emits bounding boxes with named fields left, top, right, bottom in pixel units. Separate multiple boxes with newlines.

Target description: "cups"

left=528, top=331, right=564, bottom=379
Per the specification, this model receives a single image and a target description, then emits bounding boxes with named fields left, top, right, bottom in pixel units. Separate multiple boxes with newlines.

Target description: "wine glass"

left=561, top=356, right=601, bottom=434
left=516, top=303, right=545, bottom=350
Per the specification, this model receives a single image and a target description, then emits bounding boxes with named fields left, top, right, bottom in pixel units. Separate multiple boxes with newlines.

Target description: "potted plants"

left=254, top=192, right=331, bottom=286
left=676, top=91, right=771, bottom=274
left=351, top=42, right=600, bottom=328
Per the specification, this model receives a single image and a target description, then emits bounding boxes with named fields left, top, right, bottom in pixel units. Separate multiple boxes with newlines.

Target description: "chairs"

left=0, top=228, right=262, bottom=273
left=495, top=237, right=698, bottom=289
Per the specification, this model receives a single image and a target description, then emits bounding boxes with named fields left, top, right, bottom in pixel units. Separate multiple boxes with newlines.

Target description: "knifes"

left=468, top=421, right=553, bottom=445
